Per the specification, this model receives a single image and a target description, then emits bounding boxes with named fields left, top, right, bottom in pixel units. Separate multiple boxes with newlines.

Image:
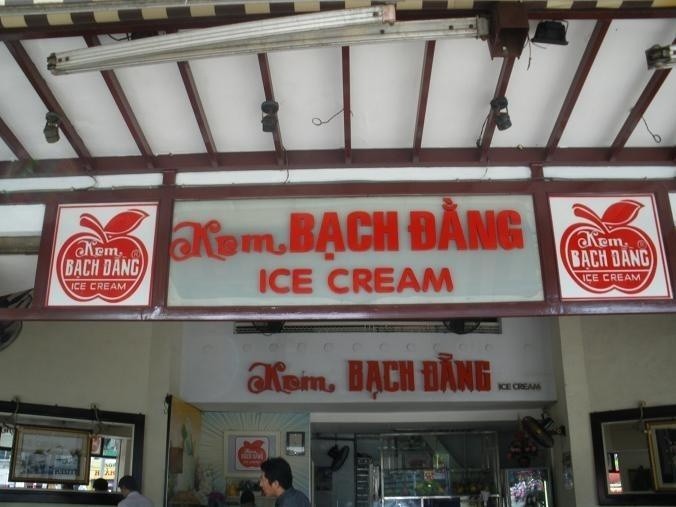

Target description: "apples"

left=55, top=208, right=150, bottom=303
left=238, top=439, right=266, bottom=467
left=559, top=199, right=657, bottom=294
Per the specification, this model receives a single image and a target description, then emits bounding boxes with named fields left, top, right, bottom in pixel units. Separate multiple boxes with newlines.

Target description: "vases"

left=519, top=453, right=531, bottom=467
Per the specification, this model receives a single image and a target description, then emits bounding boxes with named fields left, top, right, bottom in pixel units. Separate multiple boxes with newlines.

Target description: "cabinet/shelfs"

left=355, top=461, right=375, bottom=506
left=502, top=465, right=555, bottom=506
left=378, top=429, right=502, bottom=507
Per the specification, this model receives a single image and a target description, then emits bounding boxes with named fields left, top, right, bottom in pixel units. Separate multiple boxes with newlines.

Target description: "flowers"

left=504, top=430, right=542, bottom=460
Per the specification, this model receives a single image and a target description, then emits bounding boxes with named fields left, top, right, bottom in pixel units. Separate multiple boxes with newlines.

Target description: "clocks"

left=286, top=429, right=306, bottom=457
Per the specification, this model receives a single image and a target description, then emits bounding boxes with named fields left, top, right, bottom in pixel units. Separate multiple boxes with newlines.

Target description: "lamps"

left=489, top=94, right=518, bottom=132
left=40, top=109, right=66, bottom=149
left=528, top=11, right=573, bottom=50
left=259, top=97, right=282, bottom=135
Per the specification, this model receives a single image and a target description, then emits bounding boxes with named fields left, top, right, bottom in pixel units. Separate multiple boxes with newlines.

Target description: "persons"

left=240, top=490, right=256, bottom=507
left=93, top=478, right=108, bottom=491
left=118, top=475, right=154, bottom=507
left=259, top=457, right=312, bottom=507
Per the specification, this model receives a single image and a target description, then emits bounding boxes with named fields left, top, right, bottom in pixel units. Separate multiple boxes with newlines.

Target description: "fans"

left=0, top=290, right=36, bottom=327
left=519, top=409, right=569, bottom=451
left=442, top=316, right=485, bottom=333
left=323, top=432, right=352, bottom=472
left=0, top=317, right=26, bottom=355
left=247, top=319, right=287, bottom=337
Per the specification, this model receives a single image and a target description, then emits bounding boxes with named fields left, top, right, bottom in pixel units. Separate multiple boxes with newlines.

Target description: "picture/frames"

left=220, top=428, right=281, bottom=480
left=314, top=464, right=337, bottom=494
left=8, top=423, right=92, bottom=486
left=645, top=417, right=676, bottom=492
